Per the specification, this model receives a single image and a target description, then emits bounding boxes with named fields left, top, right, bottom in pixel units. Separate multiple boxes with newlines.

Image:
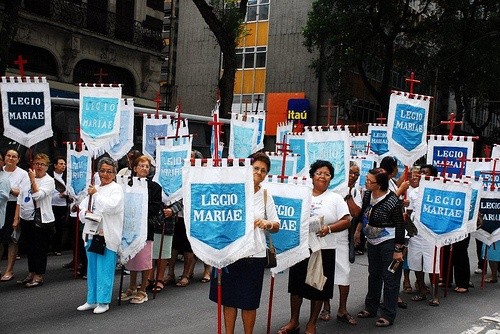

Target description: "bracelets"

left=328, top=226, right=331, bottom=233
left=10, top=189, right=13, bottom=194
left=268, top=222, right=273, bottom=230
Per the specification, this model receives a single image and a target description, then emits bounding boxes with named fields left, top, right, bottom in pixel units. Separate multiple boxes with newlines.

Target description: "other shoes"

left=17, top=275, right=33, bottom=285
left=1, top=271, right=14, bottom=280
left=278, top=269, right=500, bottom=334
left=54, top=251, right=61, bottom=255
left=26, top=279, right=44, bottom=286
left=15, top=255, right=20, bottom=259
left=77, top=302, right=96, bottom=311
left=93, top=304, right=109, bottom=313
left=355, top=250, right=364, bottom=255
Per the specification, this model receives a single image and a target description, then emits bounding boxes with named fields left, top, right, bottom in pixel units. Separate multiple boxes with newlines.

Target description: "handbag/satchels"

left=87, top=234, right=106, bottom=255
left=32, top=207, right=42, bottom=228
left=264, top=248, right=277, bottom=268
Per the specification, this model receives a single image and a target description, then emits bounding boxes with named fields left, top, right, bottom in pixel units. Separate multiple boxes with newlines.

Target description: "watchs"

left=394, top=245, right=402, bottom=252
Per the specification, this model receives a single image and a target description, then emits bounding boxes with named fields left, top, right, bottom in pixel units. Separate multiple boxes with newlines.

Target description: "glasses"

left=348, top=170, right=360, bottom=176
left=254, top=167, right=267, bottom=174
left=33, top=162, right=48, bottom=167
left=364, top=175, right=377, bottom=184
left=313, top=172, right=331, bottom=178
left=100, top=168, right=114, bottom=174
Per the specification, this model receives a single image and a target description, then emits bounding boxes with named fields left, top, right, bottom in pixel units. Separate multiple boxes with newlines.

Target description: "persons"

left=12, top=153, right=55, bottom=287
left=0, top=147, right=28, bottom=281
left=136, top=151, right=212, bottom=293
left=48, top=156, right=68, bottom=256
left=0, top=153, right=11, bottom=229
left=403, top=164, right=500, bottom=306
left=77, top=157, right=124, bottom=314
left=117, top=155, right=163, bottom=303
left=379, top=156, right=410, bottom=308
left=319, top=165, right=362, bottom=325
left=354, top=168, right=405, bottom=327
left=276, top=160, right=352, bottom=334
left=208, top=153, right=280, bottom=334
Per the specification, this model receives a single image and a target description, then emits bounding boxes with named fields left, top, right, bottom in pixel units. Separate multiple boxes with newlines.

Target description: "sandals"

left=137, top=256, right=211, bottom=292
left=130, top=291, right=148, bottom=303
left=117, top=289, right=137, bottom=300
